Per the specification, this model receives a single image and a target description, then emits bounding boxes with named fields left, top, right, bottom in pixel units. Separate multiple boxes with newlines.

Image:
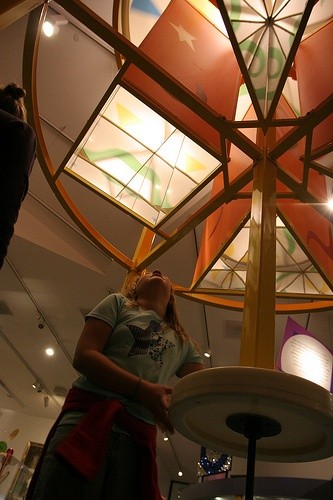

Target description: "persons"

left=25, top=269, right=205, bottom=500
left=0, top=83, right=38, bottom=271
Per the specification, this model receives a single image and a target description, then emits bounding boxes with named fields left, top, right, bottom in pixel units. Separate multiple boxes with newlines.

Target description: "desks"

left=167, top=366, right=333, bottom=500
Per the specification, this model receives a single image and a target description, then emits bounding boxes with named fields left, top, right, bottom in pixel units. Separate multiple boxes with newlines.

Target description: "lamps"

left=37, top=385, right=43, bottom=393
left=37, top=319, right=47, bottom=329
left=31, top=380, right=40, bottom=389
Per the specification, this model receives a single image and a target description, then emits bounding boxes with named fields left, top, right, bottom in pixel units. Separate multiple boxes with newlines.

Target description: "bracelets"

left=130, top=376, right=141, bottom=399
